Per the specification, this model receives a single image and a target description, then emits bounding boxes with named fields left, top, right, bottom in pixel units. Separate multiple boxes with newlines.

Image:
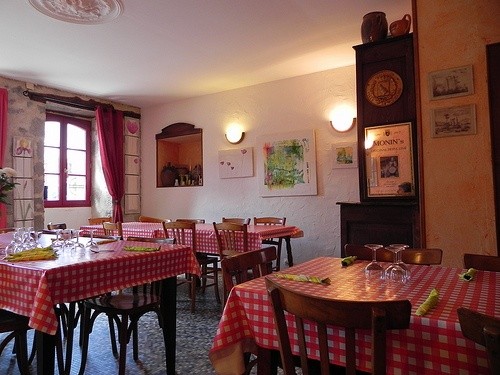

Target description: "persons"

left=396, top=182, right=411, bottom=194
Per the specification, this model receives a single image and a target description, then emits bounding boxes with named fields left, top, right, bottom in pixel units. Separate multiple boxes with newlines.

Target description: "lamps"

left=329, top=117, right=356, bottom=133
left=225, top=132, right=245, bottom=145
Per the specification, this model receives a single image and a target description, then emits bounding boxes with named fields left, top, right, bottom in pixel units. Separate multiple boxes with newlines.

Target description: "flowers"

left=0, top=167, right=22, bottom=205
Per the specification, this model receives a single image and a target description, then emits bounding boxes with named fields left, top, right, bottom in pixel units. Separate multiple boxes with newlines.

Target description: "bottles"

left=161, top=169, right=177, bottom=186
left=361, top=11, right=388, bottom=44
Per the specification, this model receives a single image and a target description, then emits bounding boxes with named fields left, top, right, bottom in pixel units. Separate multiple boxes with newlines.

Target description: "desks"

left=80, top=221, right=163, bottom=304
left=155, top=223, right=304, bottom=313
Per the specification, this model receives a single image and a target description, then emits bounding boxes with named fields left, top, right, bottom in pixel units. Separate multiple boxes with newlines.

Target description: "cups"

left=389, top=14, right=411, bottom=37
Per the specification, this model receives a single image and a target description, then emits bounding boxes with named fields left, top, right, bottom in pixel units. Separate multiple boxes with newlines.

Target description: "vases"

left=160, top=166, right=178, bottom=186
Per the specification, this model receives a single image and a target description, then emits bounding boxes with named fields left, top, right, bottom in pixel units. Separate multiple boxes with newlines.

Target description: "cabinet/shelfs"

left=336, top=31, right=422, bottom=257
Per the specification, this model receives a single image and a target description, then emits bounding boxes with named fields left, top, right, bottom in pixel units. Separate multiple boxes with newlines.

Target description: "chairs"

left=0, top=217, right=500, bottom=375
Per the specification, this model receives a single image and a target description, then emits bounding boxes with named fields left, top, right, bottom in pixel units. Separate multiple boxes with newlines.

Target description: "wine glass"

left=363, top=243, right=411, bottom=286
left=5, top=225, right=99, bottom=258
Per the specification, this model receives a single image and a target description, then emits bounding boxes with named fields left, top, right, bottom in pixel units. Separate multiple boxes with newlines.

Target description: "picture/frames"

left=428, top=64, right=475, bottom=102
left=430, top=103, right=477, bottom=138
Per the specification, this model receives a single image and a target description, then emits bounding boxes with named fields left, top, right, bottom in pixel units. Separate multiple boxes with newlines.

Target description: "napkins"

left=122, top=244, right=161, bottom=251
left=341, top=256, right=357, bottom=266
left=4, top=249, right=59, bottom=260
left=274, top=273, right=332, bottom=285
left=8, top=254, right=56, bottom=262
left=415, top=288, right=439, bottom=316
left=458, top=268, right=476, bottom=281
left=21, top=245, right=53, bottom=252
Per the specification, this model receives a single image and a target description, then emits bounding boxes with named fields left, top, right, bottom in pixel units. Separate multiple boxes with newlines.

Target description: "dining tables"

left=208, top=256, right=500, bottom=375
left=0, top=230, right=203, bottom=375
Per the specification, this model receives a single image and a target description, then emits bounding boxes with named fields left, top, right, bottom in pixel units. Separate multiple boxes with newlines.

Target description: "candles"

left=190, top=160, right=192, bottom=171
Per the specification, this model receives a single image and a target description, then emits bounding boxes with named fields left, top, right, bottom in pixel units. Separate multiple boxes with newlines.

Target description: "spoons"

left=90, top=249, right=114, bottom=253
